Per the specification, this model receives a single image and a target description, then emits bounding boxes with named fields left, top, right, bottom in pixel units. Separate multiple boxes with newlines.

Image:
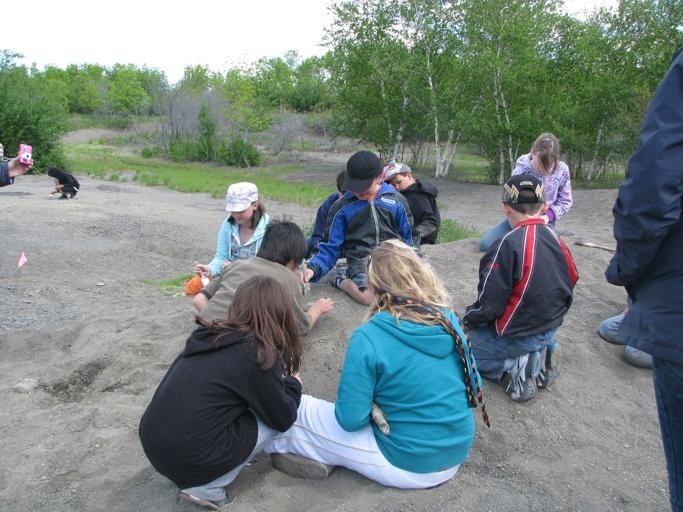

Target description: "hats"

left=225, top=151, right=412, bottom=212
left=502, top=173, right=545, bottom=205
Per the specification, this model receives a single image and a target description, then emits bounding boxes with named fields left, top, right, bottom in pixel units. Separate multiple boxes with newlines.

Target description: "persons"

left=138, top=274, right=305, bottom=512
left=602, top=52, right=683, bottom=512
left=294, top=150, right=439, bottom=306
left=478, top=132, right=574, bottom=253
left=0, top=157, right=33, bottom=187
left=462, top=174, right=580, bottom=402
left=184, top=181, right=274, bottom=296
left=261, top=238, right=491, bottom=491
left=596, top=307, right=653, bottom=370
left=47, top=167, right=80, bottom=199
left=192, top=214, right=335, bottom=335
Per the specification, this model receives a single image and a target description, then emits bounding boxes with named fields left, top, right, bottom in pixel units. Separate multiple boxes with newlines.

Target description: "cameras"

left=18, top=144, right=32, bottom=167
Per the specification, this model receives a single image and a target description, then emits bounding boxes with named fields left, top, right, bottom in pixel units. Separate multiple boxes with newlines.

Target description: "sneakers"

left=330, top=263, right=349, bottom=287
left=178, top=490, right=236, bottom=511
left=538, top=342, right=562, bottom=389
left=270, top=453, right=329, bottom=480
left=506, top=351, right=542, bottom=402
